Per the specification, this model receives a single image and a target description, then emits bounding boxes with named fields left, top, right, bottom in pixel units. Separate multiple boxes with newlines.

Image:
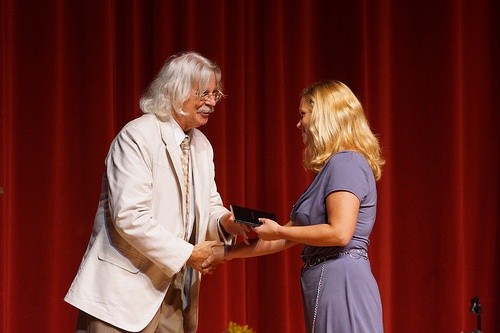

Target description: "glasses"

left=192, top=90, right=220, bottom=101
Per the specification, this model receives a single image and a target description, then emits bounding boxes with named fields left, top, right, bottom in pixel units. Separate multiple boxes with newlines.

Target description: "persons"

left=203, top=81, right=385, bottom=333
left=62, top=51, right=257, bottom=332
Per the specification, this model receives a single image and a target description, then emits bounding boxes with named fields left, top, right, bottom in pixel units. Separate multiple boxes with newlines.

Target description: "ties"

left=171, top=136, right=193, bottom=314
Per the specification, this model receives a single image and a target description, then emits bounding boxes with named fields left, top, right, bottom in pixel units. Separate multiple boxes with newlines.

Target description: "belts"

left=300, top=246, right=341, bottom=267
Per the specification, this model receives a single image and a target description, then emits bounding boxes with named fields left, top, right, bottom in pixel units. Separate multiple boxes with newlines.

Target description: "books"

left=230, top=204, right=276, bottom=227
